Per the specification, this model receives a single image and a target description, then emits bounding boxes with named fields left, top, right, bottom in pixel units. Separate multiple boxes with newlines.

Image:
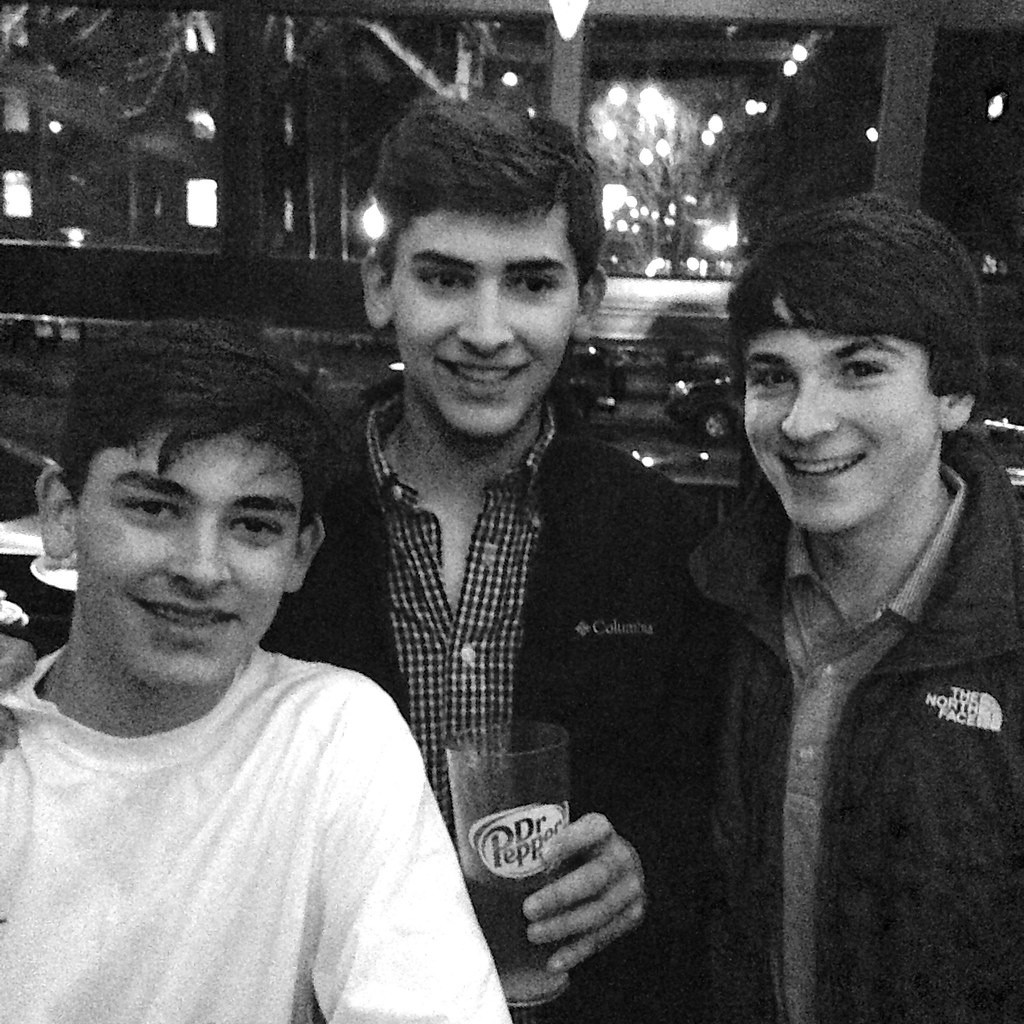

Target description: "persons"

left=2, top=95, right=718, bottom=1024
left=0, top=316, right=513, bottom=1024
left=682, top=193, right=1024, bottom=1024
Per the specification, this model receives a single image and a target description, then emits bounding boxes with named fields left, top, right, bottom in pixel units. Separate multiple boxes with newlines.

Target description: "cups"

left=445, top=718, right=580, bottom=1012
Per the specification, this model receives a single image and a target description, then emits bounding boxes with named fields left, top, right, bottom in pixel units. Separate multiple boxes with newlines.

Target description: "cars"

left=596, top=225, right=752, bottom=287
